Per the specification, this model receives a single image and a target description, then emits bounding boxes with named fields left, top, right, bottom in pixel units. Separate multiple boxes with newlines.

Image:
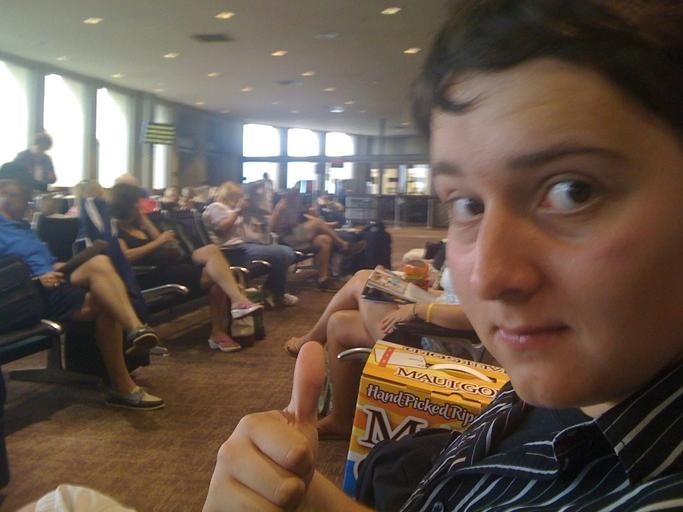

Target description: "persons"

left=270, top=181, right=366, bottom=292
left=16, top=1, right=683, bottom=510
left=67, top=173, right=271, bottom=217
left=13, top=133, right=56, bottom=192
left=284, top=238, right=485, bottom=440
left=0, top=161, right=166, bottom=411
left=202, top=182, right=298, bottom=308
left=106, top=183, right=263, bottom=352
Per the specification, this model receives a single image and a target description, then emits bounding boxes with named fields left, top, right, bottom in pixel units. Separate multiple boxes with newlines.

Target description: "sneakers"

left=124, top=324, right=160, bottom=357
left=316, top=275, right=340, bottom=292
left=342, top=240, right=367, bottom=261
left=104, top=386, right=165, bottom=410
left=266, top=294, right=298, bottom=308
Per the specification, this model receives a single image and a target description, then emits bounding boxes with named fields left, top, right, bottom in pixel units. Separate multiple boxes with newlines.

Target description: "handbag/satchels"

left=0, top=254, right=48, bottom=333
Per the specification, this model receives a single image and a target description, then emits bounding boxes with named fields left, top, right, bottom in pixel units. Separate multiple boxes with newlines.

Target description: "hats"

left=1, top=162, right=48, bottom=192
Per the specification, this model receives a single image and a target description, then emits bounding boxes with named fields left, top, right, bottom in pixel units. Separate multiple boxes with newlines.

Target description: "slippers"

left=231, top=299, right=264, bottom=319
left=283, top=337, right=300, bottom=358
left=208, top=331, right=242, bottom=352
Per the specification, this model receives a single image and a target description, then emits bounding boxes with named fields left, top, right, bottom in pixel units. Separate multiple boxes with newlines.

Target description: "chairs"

left=0, top=176, right=487, bottom=410
